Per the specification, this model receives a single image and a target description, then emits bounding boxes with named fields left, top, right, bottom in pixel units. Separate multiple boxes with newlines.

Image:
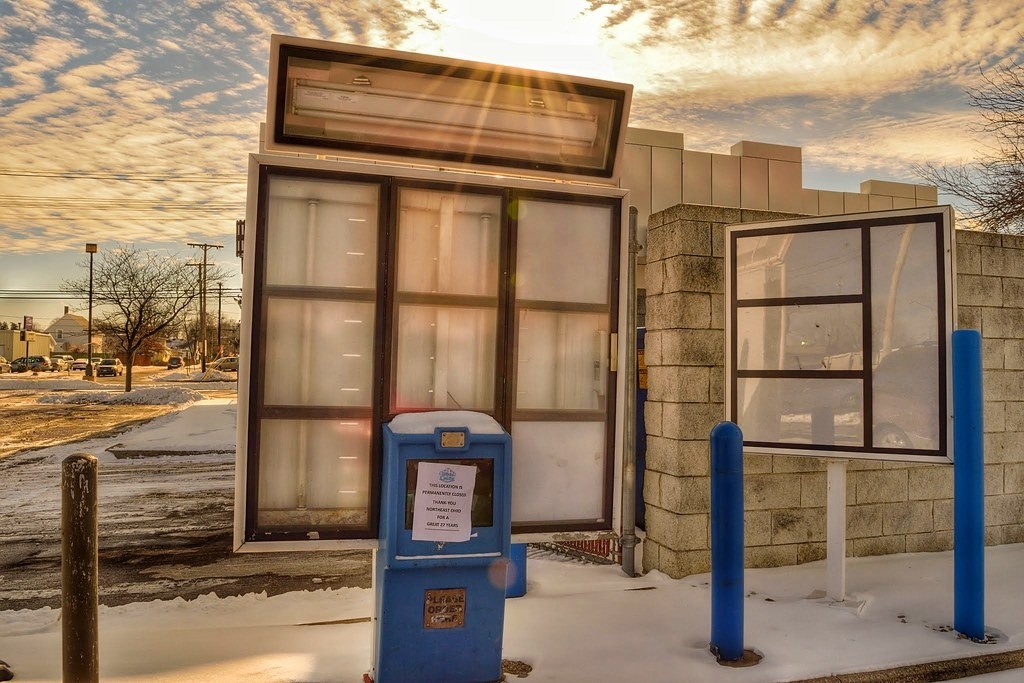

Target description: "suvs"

left=10, top=355, right=52, bottom=372
left=168, top=357, right=185, bottom=370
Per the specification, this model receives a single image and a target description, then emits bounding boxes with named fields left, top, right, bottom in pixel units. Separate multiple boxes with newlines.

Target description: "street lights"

left=85, top=244, right=97, bottom=376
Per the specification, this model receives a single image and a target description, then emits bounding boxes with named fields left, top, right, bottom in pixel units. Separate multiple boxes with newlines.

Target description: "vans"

left=52, top=355, right=74, bottom=369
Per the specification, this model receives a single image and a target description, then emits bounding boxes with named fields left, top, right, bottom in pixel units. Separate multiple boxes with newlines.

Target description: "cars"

left=91, top=357, right=102, bottom=364
left=50, top=357, right=70, bottom=372
left=0, top=355, right=12, bottom=374
left=206, top=357, right=238, bottom=371
left=97, top=358, right=124, bottom=377
left=72, top=358, right=96, bottom=371
